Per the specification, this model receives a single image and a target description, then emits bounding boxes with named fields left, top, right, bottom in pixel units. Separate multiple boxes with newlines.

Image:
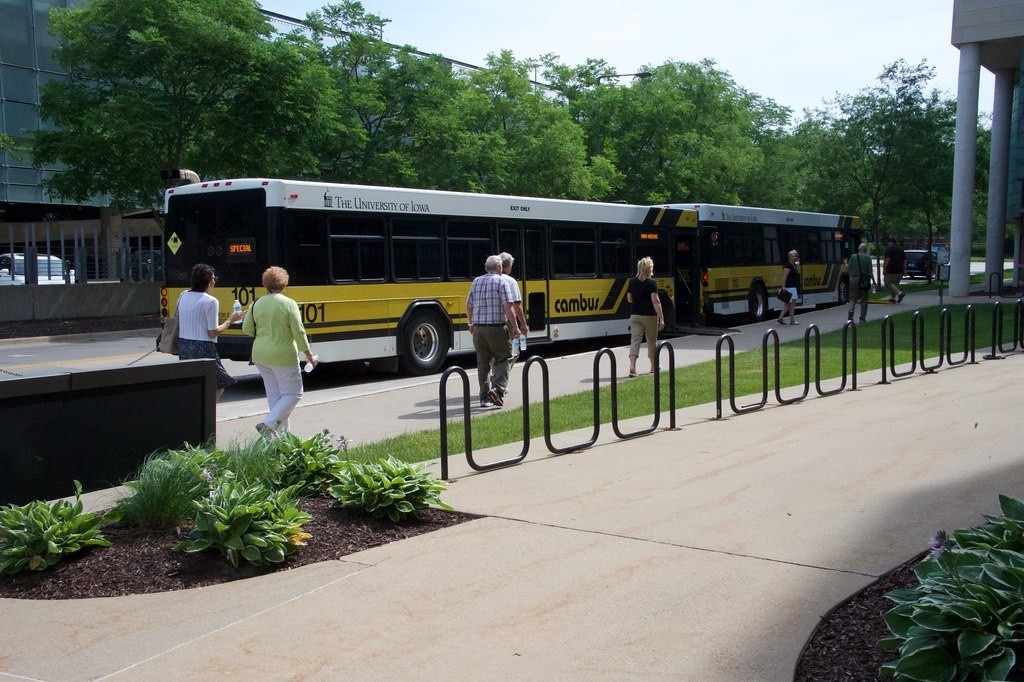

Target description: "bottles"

left=512, top=337, right=519, bottom=356
left=304, top=354, right=319, bottom=373
left=233, top=300, right=242, bottom=324
left=872, top=285, right=876, bottom=293
left=519, top=333, right=526, bottom=350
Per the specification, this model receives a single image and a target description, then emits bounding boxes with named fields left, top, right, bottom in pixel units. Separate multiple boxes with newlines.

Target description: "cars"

left=133, top=250, right=162, bottom=282
left=903, top=249, right=933, bottom=279
left=0, top=253, right=75, bottom=286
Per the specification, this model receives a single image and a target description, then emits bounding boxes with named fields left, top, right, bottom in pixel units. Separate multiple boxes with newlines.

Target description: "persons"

left=626, top=256, right=665, bottom=379
left=842, top=242, right=877, bottom=324
left=777, top=249, right=801, bottom=326
left=882, top=236, right=908, bottom=302
left=174, top=263, right=249, bottom=406
left=242, top=266, right=320, bottom=445
left=465, top=251, right=531, bottom=408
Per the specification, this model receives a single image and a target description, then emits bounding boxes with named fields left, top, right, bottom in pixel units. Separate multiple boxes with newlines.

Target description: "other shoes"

left=889, top=298, right=896, bottom=304
left=860, top=318, right=866, bottom=323
left=848, top=310, right=854, bottom=321
left=897, top=292, right=905, bottom=303
left=790, top=320, right=799, bottom=325
left=255, top=423, right=277, bottom=441
left=777, top=319, right=786, bottom=325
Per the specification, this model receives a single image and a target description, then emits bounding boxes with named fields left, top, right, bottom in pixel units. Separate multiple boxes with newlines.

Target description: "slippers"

left=650, top=367, right=661, bottom=372
left=629, top=373, right=638, bottom=377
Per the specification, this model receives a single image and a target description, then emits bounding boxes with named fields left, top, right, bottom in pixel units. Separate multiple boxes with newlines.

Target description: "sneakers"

left=480, top=400, right=494, bottom=406
left=488, top=388, right=504, bottom=406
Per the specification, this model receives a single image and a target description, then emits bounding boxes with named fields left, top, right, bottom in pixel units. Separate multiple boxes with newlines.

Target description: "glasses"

left=211, top=277, right=217, bottom=282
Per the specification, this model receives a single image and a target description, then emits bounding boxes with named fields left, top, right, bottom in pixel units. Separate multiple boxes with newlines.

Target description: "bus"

left=647, top=202, right=877, bottom=323
left=156, top=169, right=720, bottom=377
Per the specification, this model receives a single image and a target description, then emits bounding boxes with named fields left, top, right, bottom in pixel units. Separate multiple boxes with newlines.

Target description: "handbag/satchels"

left=858, top=275, right=871, bottom=290
left=776, top=288, right=792, bottom=303
left=158, top=292, right=179, bottom=354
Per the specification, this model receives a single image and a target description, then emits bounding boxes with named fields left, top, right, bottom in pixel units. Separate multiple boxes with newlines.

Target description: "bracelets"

left=224, top=321, right=232, bottom=326
left=467, top=320, right=474, bottom=327
left=306, top=353, right=315, bottom=359
